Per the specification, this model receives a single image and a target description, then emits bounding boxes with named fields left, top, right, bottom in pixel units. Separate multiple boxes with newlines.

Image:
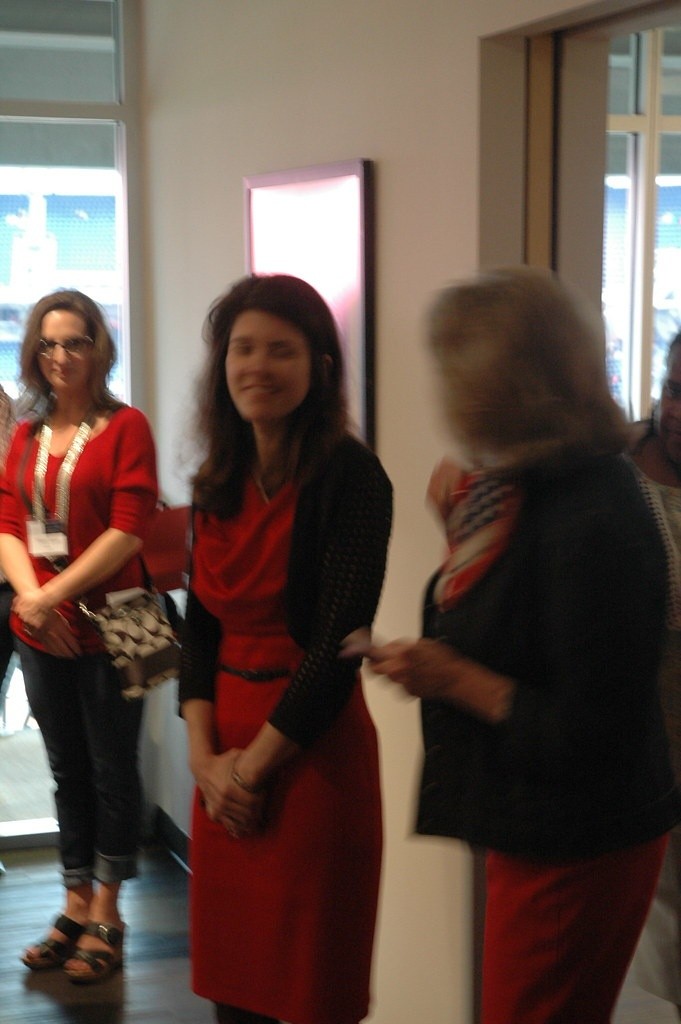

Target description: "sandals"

left=17, top=908, right=127, bottom=985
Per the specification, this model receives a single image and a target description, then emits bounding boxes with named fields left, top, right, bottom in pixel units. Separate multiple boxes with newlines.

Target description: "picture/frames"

left=242, top=158, right=375, bottom=450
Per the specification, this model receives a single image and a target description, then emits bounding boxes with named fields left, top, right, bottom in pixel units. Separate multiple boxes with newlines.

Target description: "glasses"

left=33, top=333, right=98, bottom=360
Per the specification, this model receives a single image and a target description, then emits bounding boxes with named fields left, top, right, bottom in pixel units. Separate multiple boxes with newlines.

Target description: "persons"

left=176, top=275, right=395, bottom=1024
left=345, top=266, right=681, bottom=1024
left=0, top=291, right=159, bottom=983
left=613, top=332, right=681, bottom=1005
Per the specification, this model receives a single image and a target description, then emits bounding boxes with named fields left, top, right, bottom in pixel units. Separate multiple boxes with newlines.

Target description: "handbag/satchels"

left=78, top=584, right=185, bottom=702
